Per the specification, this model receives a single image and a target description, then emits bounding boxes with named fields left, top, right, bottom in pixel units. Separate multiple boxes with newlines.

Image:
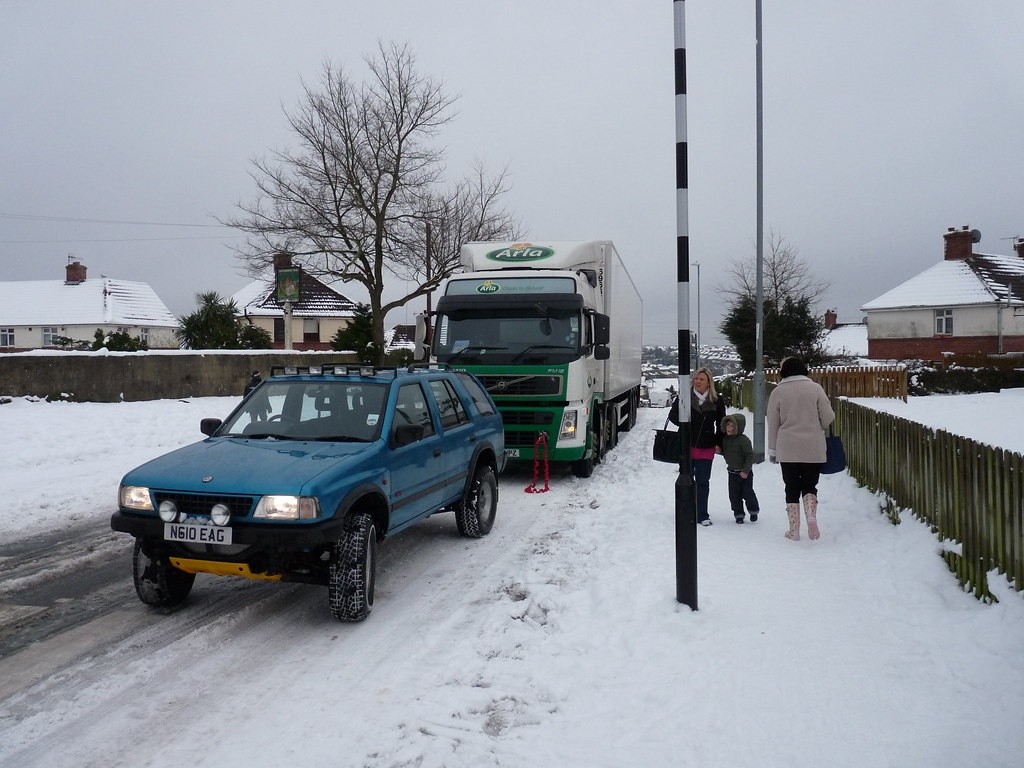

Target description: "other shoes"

left=699, top=519, right=712, bottom=526
left=750, top=514, right=757, bottom=521
left=736, top=518, right=743, bottom=524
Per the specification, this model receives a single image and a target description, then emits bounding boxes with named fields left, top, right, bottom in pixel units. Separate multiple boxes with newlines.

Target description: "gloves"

left=770, top=455, right=779, bottom=464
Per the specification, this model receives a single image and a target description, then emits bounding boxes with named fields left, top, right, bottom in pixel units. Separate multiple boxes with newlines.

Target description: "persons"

left=767, top=356, right=837, bottom=541
left=714, top=414, right=760, bottom=523
left=668, top=367, right=727, bottom=526
left=243, top=370, right=272, bottom=423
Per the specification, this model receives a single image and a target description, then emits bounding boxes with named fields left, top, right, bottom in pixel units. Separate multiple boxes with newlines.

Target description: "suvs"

left=109, top=363, right=507, bottom=624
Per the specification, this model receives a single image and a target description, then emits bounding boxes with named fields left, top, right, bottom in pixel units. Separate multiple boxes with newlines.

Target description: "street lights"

left=692, top=262, right=701, bottom=370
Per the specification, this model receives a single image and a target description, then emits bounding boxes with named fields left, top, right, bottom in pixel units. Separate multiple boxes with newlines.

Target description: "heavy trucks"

left=415, top=240, right=644, bottom=477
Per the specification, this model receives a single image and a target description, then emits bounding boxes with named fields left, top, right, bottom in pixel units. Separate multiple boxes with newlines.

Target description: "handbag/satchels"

left=652, top=416, right=682, bottom=464
left=818, top=425, right=845, bottom=474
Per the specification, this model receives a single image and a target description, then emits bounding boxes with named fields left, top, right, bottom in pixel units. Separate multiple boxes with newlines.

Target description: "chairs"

left=299, top=391, right=341, bottom=438
left=352, top=391, right=401, bottom=442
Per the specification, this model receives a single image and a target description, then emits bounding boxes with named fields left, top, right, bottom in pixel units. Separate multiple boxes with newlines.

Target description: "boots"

left=785, top=503, right=801, bottom=541
left=802, top=493, right=820, bottom=540
